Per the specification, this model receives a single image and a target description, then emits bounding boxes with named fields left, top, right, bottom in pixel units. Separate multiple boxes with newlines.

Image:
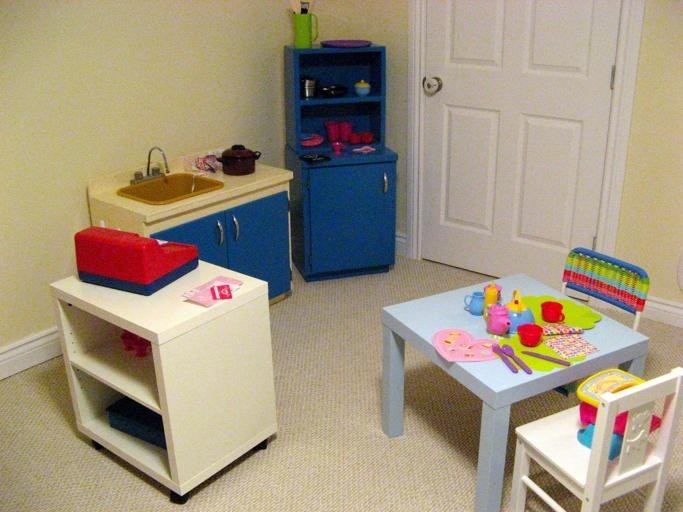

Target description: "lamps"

left=509, top=366, right=683, bottom=512
left=552, top=247, right=650, bottom=396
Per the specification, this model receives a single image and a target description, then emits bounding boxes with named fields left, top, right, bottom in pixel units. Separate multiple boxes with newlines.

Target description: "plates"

left=144, top=173, right=293, bottom=306
left=51, top=259, right=278, bottom=504
left=285, top=46, right=398, bottom=283
